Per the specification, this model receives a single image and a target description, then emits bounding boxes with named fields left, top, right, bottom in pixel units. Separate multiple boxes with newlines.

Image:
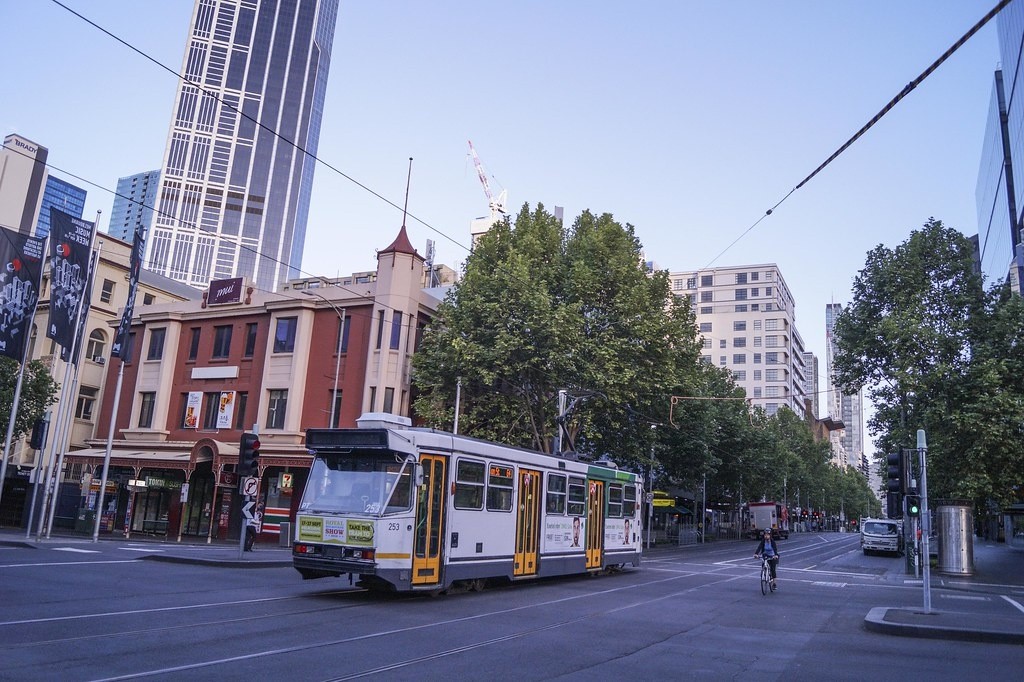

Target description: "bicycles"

left=758, top=556, right=774, bottom=595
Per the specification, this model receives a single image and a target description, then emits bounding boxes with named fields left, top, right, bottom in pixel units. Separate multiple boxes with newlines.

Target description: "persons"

left=570, top=517, right=581, bottom=547
left=623, top=519, right=630, bottom=545
left=754, top=528, right=780, bottom=589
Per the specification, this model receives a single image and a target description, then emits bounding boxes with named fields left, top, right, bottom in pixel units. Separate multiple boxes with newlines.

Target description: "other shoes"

left=773, top=583, right=777, bottom=590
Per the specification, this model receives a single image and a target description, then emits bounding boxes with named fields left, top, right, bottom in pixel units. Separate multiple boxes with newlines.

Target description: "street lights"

left=302, top=289, right=346, bottom=429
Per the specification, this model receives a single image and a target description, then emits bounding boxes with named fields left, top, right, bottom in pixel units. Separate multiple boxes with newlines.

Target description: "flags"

left=0, top=222, right=48, bottom=365
left=60, top=250, right=97, bottom=367
left=109, top=229, right=146, bottom=359
left=46, top=205, right=95, bottom=354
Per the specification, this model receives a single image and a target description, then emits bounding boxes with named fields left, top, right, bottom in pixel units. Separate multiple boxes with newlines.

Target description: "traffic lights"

left=238, top=432, right=261, bottom=476
left=887, top=444, right=905, bottom=492
left=905, top=487, right=923, bottom=517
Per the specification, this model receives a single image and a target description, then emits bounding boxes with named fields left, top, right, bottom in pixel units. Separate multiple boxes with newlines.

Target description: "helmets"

left=764, top=528, right=772, bottom=534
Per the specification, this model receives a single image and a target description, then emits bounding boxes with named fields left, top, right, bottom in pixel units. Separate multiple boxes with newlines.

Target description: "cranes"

left=464, top=138, right=509, bottom=219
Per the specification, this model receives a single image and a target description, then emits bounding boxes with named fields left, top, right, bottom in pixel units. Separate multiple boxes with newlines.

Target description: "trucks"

left=860, top=517, right=903, bottom=558
left=747, top=502, right=792, bottom=542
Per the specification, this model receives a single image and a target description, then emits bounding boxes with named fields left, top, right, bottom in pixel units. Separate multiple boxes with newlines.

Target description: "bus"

left=292, top=395, right=655, bottom=600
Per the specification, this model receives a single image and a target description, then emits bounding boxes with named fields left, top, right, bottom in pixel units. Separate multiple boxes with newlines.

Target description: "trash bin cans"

left=72, top=508, right=97, bottom=537
left=279, top=522, right=296, bottom=548
